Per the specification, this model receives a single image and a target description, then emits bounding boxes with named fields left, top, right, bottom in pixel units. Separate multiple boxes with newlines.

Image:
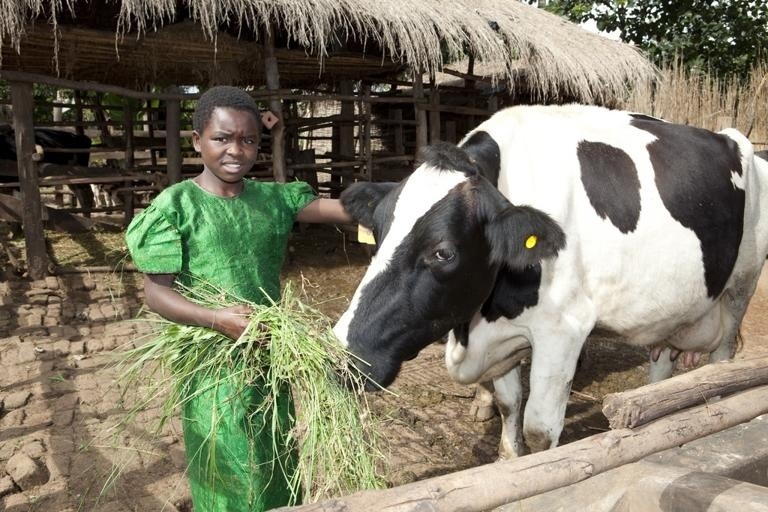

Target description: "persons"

left=125, top=85, right=354, bottom=511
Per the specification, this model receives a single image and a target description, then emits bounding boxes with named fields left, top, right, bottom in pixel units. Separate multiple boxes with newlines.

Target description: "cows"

left=331, top=103, right=768, bottom=463
left=0, top=124, right=200, bottom=221
